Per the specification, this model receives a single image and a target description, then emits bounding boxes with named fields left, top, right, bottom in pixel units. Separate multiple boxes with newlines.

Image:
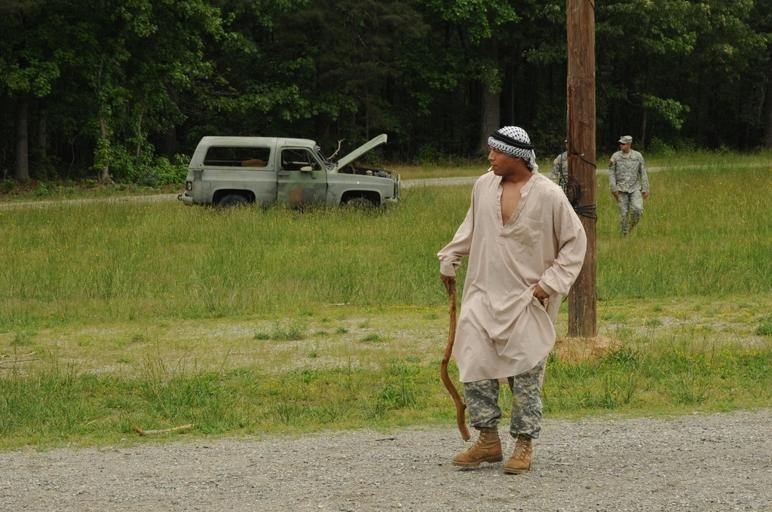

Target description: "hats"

left=618, top=135, right=633, bottom=144
left=487, top=125, right=536, bottom=160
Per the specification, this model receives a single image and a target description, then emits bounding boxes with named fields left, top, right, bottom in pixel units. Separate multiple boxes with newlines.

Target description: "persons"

left=552, top=139, right=568, bottom=189
left=439, top=125, right=588, bottom=472
left=608, top=134, right=650, bottom=238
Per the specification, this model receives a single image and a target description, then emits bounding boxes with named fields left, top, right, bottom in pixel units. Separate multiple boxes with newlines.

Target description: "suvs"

left=175, top=132, right=400, bottom=212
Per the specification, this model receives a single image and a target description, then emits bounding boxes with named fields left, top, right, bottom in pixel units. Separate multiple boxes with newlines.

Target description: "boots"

left=451, top=428, right=503, bottom=467
left=503, top=434, right=533, bottom=476
